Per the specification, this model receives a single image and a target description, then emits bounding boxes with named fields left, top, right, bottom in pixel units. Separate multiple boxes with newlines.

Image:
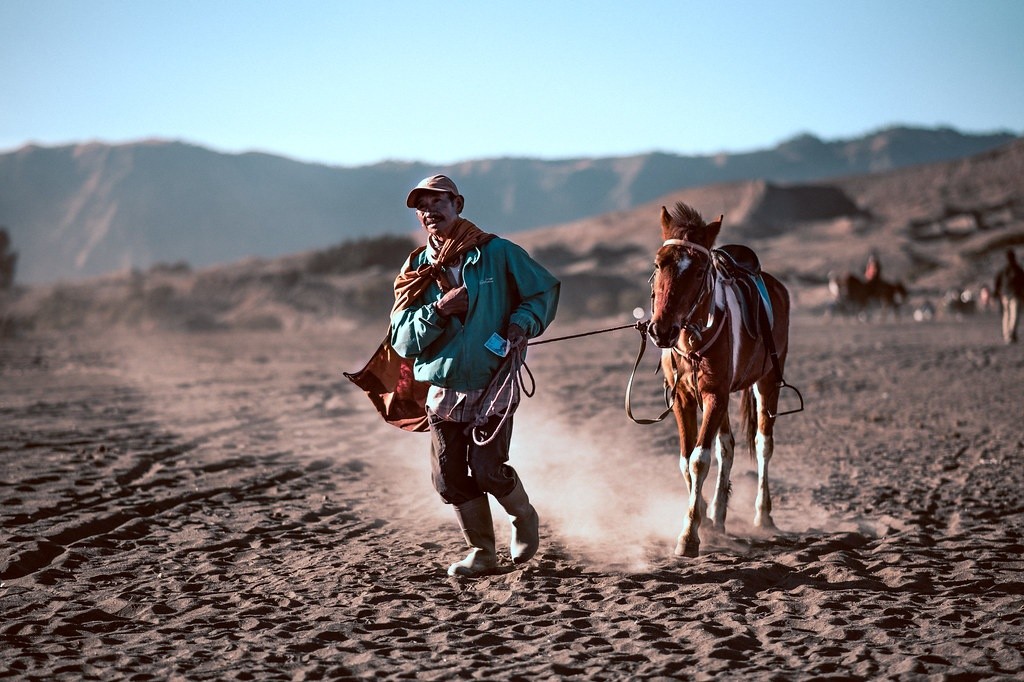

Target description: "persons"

left=993, top=249, right=1024, bottom=347
left=342, top=175, right=560, bottom=578
left=864, top=249, right=881, bottom=285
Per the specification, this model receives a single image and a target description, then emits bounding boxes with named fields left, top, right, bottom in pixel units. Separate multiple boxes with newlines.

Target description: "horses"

left=839, top=268, right=911, bottom=321
left=644, top=204, right=792, bottom=556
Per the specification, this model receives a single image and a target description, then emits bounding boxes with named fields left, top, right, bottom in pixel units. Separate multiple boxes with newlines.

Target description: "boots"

left=494, top=475, right=539, bottom=564
left=447, top=492, right=497, bottom=578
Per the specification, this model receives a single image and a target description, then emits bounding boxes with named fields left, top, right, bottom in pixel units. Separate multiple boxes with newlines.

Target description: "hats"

left=406, top=174, right=458, bottom=209
